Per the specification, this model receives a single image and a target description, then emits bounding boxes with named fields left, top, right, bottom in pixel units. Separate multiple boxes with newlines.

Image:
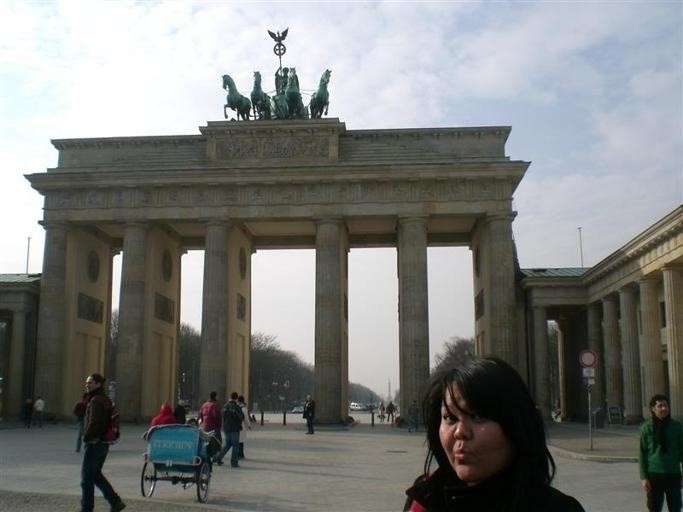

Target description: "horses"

left=251, top=71, right=271, bottom=121
left=222, top=75, right=251, bottom=122
left=283, top=67, right=303, bottom=119
left=310, top=69, right=332, bottom=118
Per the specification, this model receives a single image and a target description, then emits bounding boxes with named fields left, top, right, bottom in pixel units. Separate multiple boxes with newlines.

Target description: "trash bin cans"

left=591, top=408, right=604, bottom=429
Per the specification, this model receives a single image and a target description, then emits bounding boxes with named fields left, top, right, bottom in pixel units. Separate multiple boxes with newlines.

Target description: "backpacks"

left=91, top=396, right=121, bottom=445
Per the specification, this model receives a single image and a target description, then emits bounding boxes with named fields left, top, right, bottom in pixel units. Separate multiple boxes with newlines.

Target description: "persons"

left=149, top=400, right=177, bottom=426
left=635, top=394, right=682, bottom=512
left=273, top=66, right=289, bottom=95
left=302, top=393, right=316, bottom=435
left=20, top=398, right=33, bottom=429
left=199, top=390, right=224, bottom=465
left=78, top=371, right=127, bottom=512
left=236, top=395, right=252, bottom=459
left=173, top=398, right=187, bottom=425
left=209, top=392, right=241, bottom=468
left=399, top=335, right=586, bottom=511
left=70, top=392, right=89, bottom=453
left=31, top=395, right=45, bottom=429
left=385, top=399, right=396, bottom=423
left=376, top=398, right=386, bottom=423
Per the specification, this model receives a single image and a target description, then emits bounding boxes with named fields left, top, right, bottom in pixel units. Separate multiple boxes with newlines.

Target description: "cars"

left=290, top=401, right=377, bottom=414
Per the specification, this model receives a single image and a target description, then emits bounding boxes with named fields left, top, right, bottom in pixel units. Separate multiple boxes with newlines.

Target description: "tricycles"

left=138, top=425, right=221, bottom=506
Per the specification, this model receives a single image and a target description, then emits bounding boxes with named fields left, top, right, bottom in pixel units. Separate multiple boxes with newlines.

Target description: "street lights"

left=26, top=234, right=31, bottom=275
left=544, top=312, right=568, bottom=423
left=576, top=226, right=583, bottom=269
